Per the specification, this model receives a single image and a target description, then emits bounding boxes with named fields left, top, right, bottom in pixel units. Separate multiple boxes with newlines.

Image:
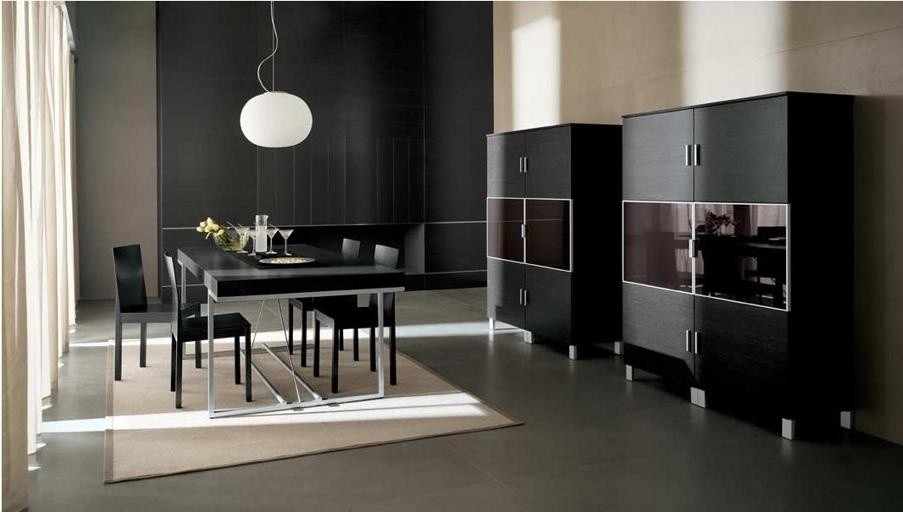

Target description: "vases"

left=214, top=225, right=249, bottom=254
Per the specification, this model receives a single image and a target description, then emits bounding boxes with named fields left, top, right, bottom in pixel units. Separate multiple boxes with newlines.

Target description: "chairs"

left=287, top=236, right=362, bottom=368
left=110, top=240, right=202, bottom=391
left=161, top=249, right=253, bottom=411
left=313, top=243, right=400, bottom=394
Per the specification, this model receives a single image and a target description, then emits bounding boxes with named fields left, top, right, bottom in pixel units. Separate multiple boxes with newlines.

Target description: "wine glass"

left=234, top=226, right=260, bottom=256
left=278, top=229, right=294, bottom=256
left=265, top=228, right=279, bottom=255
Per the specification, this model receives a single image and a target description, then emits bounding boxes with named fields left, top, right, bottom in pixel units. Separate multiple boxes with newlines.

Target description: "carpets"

left=101, top=323, right=527, bottom=487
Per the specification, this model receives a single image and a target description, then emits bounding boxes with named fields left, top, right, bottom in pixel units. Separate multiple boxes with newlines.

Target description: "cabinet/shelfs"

left=619, top=91, right=859, bottom=445
left=484, top=123, right=623, bottom=364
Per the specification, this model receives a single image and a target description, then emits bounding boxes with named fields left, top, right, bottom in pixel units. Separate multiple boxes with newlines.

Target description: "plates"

left=258, top=257, right=316, bottom=265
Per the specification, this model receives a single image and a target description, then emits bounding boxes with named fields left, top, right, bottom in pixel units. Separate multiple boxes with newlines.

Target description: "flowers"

left=196, top=215, right=234, bottom=249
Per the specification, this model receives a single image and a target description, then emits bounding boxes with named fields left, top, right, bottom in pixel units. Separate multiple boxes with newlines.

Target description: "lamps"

left=237, top=3, right=313, bottom=149
